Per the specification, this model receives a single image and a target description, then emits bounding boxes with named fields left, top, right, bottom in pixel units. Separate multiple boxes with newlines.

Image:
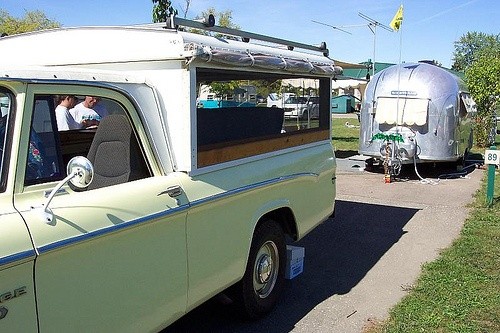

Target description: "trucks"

left=0, top=15, right=344, bottom=333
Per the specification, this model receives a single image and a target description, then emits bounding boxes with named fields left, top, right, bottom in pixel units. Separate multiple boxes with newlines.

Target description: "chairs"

left=86, top=115, right=132, bottom=191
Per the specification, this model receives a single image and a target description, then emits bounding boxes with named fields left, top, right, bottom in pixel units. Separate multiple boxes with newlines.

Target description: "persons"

left=356, top=102, right=360, bottom=111
left=55, top=94, right=87, bottom=131
left=69, top=95, right=103, bottom=129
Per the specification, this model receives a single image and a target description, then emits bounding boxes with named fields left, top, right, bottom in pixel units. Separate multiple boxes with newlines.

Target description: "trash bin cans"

left=331, top=95, right=355, bottom=114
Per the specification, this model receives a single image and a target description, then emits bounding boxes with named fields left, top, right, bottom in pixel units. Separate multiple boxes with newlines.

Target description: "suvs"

left=283, top=96, right=318, bottom=120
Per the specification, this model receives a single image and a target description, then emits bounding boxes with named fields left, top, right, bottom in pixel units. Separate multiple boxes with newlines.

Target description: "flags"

left=390, top=4, right=403, bottom=33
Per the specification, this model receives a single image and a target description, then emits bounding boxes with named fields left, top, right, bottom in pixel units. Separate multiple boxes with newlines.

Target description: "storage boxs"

left=286, top=245, right=305, bottom=280
